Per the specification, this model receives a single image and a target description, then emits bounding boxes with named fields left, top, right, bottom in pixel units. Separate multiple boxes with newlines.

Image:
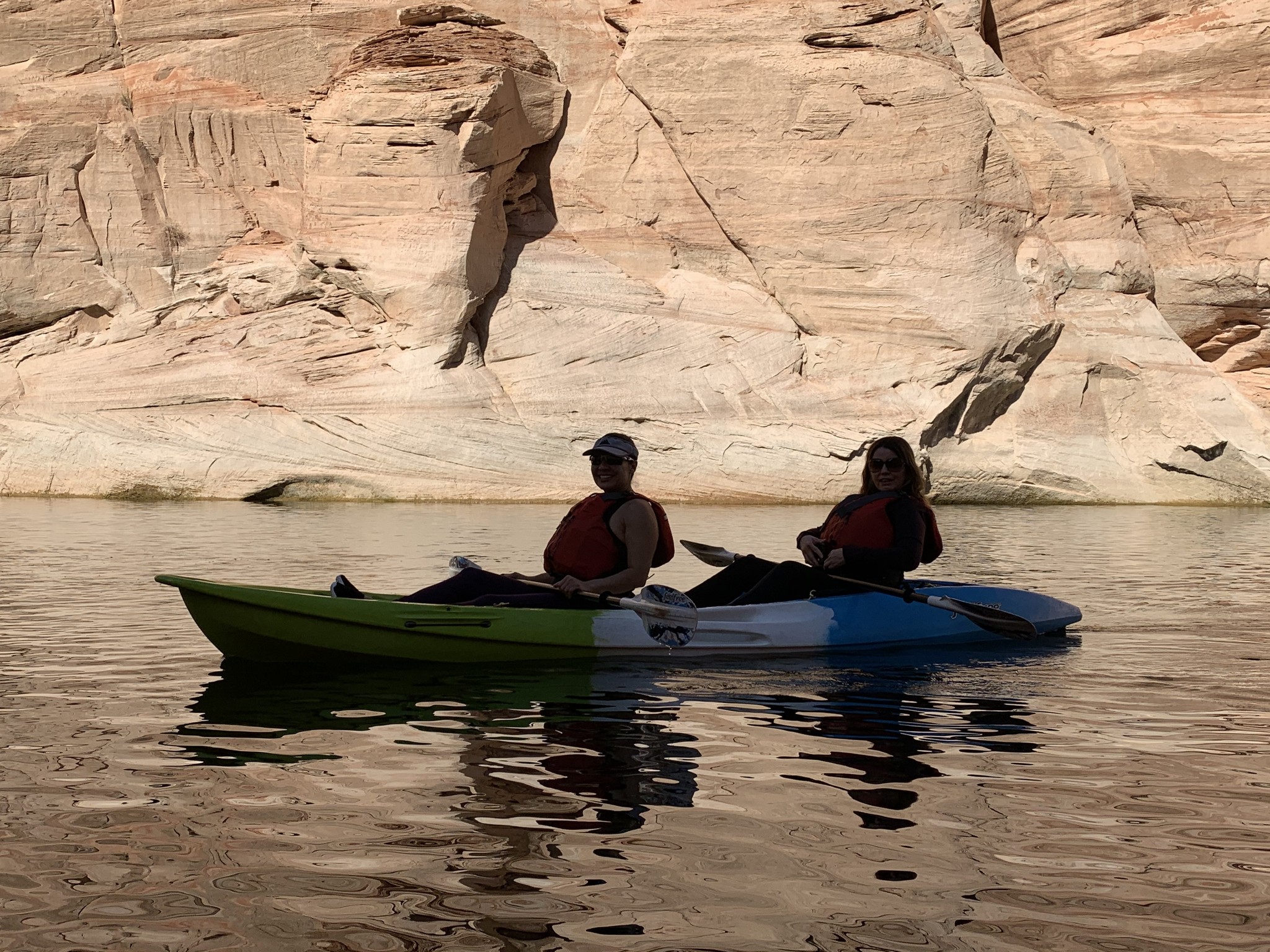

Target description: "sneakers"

left=331, top=574, right=364, bottom=599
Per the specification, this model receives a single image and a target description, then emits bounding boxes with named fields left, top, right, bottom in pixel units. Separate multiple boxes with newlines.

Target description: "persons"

left=684, top=437, right=926, bottom=609
left=331, top=433, right=658, bottom=609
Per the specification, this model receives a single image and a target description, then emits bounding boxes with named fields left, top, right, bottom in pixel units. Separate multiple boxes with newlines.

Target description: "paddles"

left=679, top=538, right=1040, bottom=642
left=446, top=554, right=700, bottom=649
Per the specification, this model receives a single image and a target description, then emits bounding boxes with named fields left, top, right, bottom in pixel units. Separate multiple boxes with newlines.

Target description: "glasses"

left=868, top=456, right=911, bottom=473
left=589, top=453, right=634, bottom=465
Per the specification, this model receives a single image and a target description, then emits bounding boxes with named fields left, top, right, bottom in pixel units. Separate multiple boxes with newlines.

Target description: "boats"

left=150, top=569, right=1089, bottom=674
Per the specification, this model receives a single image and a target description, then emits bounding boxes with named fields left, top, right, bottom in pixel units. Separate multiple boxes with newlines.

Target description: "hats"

left=582, top=433, right=638, bottom=460
